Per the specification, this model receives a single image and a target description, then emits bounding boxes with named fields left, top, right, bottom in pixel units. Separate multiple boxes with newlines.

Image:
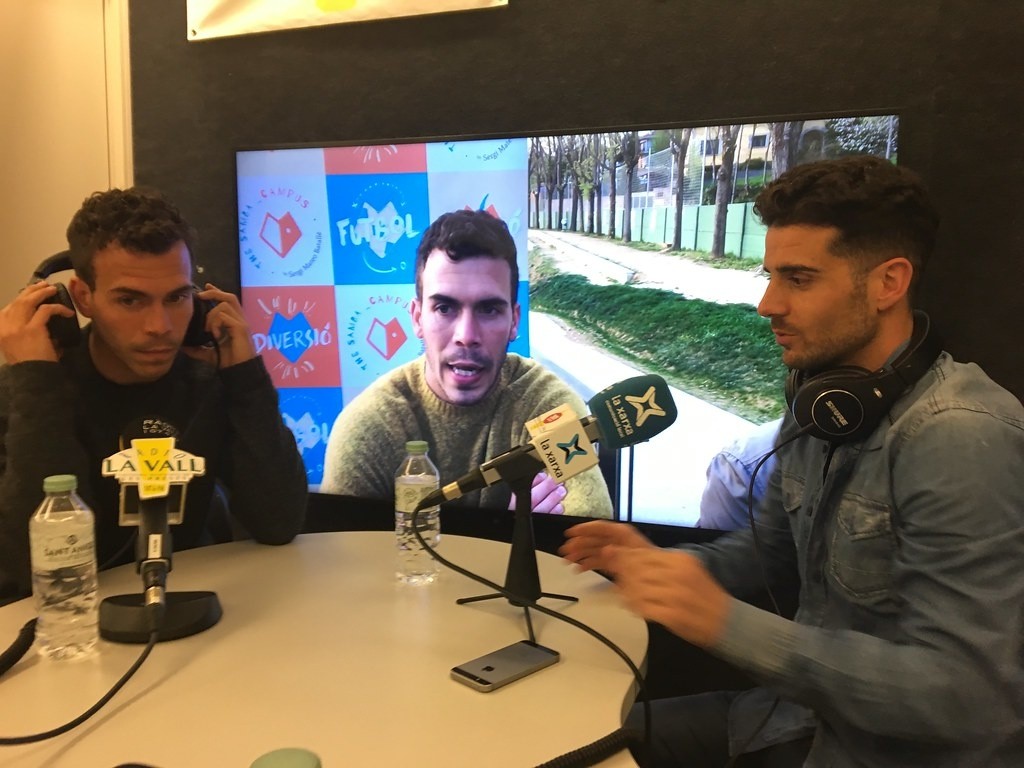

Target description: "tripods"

left=455, top=443, right=581, bottom=645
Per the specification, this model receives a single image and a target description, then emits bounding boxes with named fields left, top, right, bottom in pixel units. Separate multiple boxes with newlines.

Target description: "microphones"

left=419, top=375, right=678, bottom=511
left=101, top=418, right=205, bottom=632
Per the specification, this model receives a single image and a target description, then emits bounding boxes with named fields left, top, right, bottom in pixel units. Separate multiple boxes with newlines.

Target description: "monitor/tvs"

left=232, top=107, right=911, bottom=538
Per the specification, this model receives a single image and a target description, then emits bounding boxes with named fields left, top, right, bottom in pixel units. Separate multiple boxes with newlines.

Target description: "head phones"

left=27, top=249, right=216, bottom=349
left=784, top=307, right=944, bottom=445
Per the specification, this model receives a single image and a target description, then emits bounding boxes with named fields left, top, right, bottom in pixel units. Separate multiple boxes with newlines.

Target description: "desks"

left=1, top=532, right=645, bottom=768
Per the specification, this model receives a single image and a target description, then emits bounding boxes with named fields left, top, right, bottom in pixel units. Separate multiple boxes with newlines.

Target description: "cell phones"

left=452, top=639, right=562, bottom=692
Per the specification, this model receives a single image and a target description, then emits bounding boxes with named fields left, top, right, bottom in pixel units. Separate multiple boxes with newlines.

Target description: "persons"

left=317, top=209, right=614, bottom=521
left=558, top=159, right=1024, bottom=768
left=0, top=188, right=308, bottom=607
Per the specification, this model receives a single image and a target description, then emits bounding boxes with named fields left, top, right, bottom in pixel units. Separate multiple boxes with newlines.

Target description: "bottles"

left=394, top=440, right=441, bottom=585
left=27, top=473, right=100, bottom=662
